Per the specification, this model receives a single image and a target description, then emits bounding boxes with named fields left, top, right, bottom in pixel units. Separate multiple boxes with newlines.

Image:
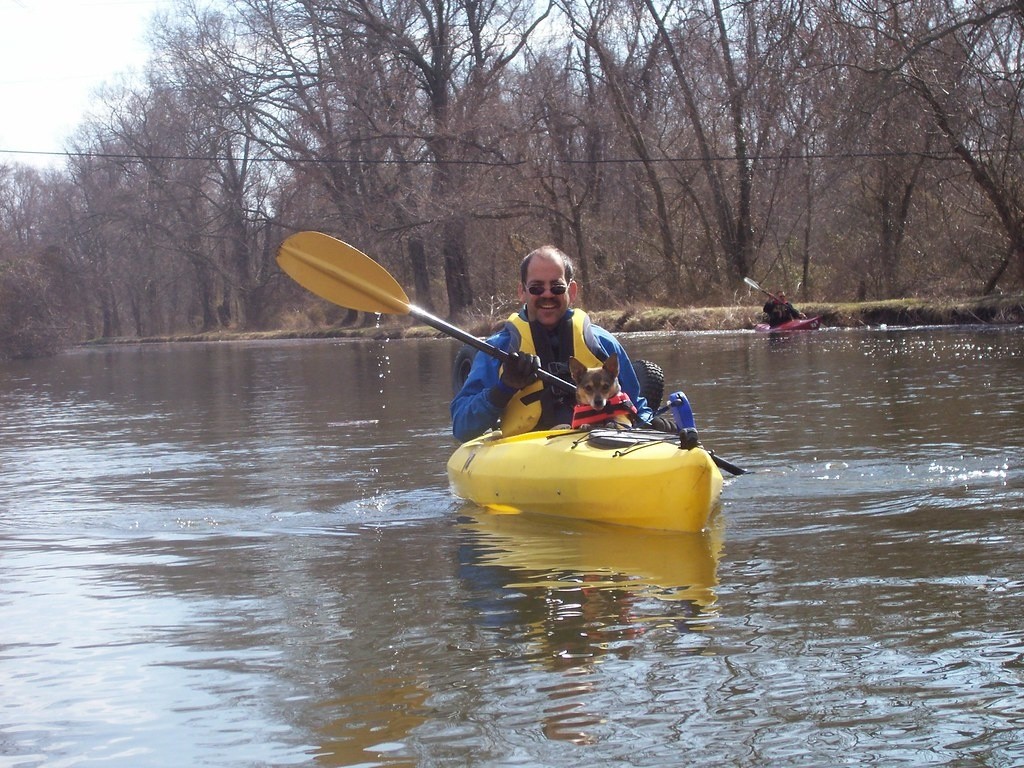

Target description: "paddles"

left=275, top=227, right=747, bottom=477
left=743, top=276, right=827, bottom=329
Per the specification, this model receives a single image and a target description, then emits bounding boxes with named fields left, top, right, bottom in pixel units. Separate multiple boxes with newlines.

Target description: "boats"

left=749, top=313, right=824, bottom=333
left=446, top=419, right=724, bottom=535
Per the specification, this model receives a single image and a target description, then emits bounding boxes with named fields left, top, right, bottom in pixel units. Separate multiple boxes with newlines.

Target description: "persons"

left=763, top=291, right=807, bottom=328
left=448, top=246, right=681, bottom=443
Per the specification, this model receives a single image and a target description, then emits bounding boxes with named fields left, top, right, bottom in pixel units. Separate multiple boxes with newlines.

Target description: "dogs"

left=548, top=352, right=638, bottom=431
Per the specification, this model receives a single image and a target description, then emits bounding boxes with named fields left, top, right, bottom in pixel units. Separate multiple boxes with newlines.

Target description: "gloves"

left=499, top=351, right=542, bottom=389
left=651, top=417, right=678, bottom=433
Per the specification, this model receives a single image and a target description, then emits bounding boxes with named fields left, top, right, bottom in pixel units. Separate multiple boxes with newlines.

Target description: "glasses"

left=778, top=295, right=785, bottom=297
left=522, top=278, right=572, bottom=295
